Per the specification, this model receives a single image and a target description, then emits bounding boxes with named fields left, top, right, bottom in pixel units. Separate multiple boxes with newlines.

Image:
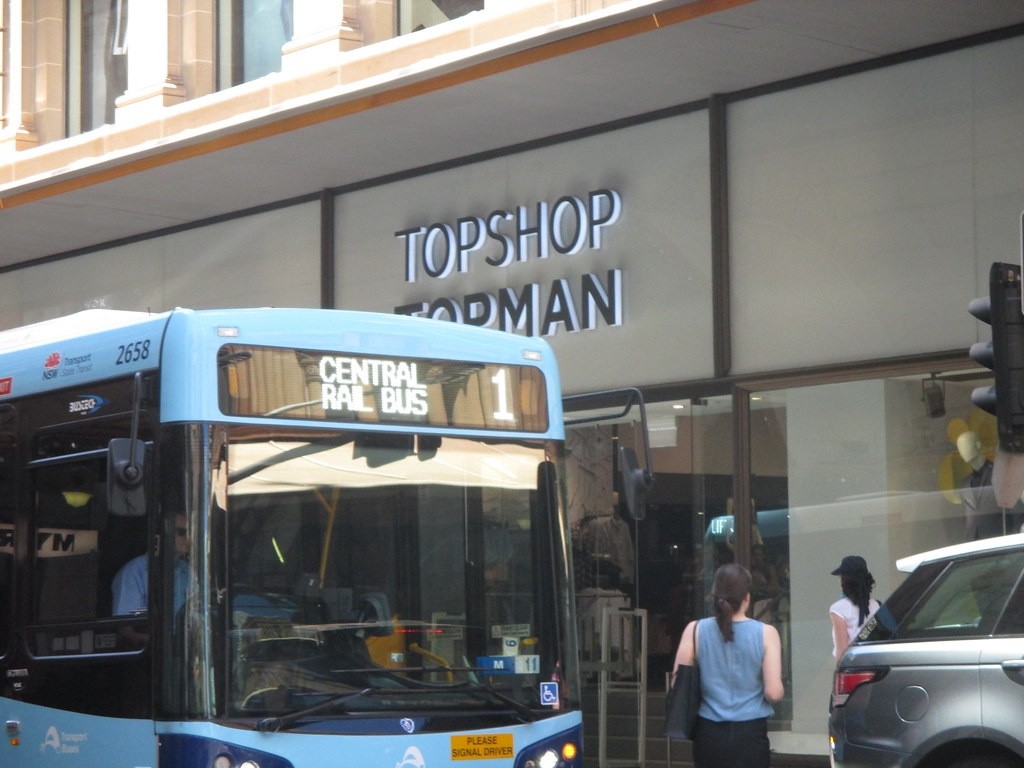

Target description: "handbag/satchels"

left=661, top=663, right=702, bottom=739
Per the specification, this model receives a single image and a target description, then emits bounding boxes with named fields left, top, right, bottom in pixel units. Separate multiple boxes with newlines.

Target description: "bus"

left=0, top=307, right=652, bottom=767
left=703, top=487, right=1024, bottom=728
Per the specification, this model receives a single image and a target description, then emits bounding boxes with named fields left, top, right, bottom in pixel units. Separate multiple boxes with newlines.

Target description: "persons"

left=111, top=507, right=295, bottom=655
left=750, top=545, right=780, bottom=596
left=830, top=556, right=882, bottom=664
left=671, top=563, right=784, bottom=768
left=957, top=431, right=1024, bottom=543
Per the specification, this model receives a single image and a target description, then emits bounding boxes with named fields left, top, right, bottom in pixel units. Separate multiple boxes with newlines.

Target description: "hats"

left=830, top=554, right=869, bottom=576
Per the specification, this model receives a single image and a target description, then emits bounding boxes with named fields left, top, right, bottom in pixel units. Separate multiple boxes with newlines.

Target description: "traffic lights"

left=967, top=298, right=997, bottom=418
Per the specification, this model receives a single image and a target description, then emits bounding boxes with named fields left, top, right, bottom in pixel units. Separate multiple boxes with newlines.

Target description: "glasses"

left=175, top=525, right=187, bottom=537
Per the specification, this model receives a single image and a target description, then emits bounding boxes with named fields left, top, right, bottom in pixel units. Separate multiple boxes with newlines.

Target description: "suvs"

left=828, top=545, right=1024, bottom=768
